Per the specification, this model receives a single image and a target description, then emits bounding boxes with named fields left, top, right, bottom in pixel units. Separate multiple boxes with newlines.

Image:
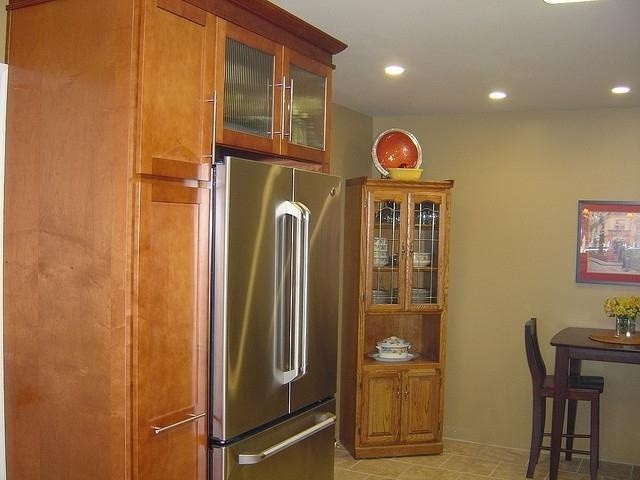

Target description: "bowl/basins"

left=373, top=238, right=390, bottom=268
left=376, top=336, right=411, bottom=359
left=388, top=167, right=424, bottom=181
left=413, top=253, right=431, bottom=268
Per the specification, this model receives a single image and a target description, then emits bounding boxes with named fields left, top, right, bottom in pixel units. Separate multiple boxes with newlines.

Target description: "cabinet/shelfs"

left=340, top=357, right=445, bottom=459
left=4, top=182, right=209, bottom=480
left=342, top=176, right=453, bottom=316
left=6, top=1, right=216, bottom=188
left=215, top=0, right=347, bottom=175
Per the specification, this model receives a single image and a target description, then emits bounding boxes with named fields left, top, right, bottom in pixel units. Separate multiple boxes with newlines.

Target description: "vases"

left=616, top=315, right=635, bottom=337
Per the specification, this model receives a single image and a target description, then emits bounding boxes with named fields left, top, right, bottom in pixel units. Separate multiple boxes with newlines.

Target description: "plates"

left=367, top=352, right=420, bottom=362
left=371, top=288, right=436, bottom=305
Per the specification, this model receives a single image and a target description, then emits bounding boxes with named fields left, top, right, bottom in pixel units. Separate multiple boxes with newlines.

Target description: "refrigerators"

left=209, top=155, right=343, bottom=480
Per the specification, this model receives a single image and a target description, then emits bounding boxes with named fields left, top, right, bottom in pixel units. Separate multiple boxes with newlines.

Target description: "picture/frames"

left=576, top=200, right=640, bottom=286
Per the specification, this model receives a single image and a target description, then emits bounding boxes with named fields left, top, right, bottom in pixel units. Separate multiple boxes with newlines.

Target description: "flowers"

left=604, top=296, right=640, bottom=317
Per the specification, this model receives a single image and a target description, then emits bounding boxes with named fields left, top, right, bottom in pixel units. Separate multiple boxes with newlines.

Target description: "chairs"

left=525, top=318, right=603, bottom=480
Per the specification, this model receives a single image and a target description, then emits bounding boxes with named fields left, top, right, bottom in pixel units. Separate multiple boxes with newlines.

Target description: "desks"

left=551, top=327, right=640, bottom=480
